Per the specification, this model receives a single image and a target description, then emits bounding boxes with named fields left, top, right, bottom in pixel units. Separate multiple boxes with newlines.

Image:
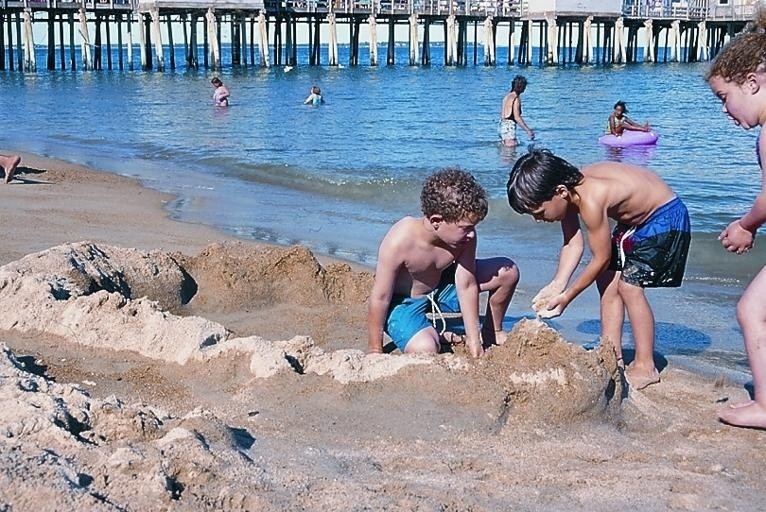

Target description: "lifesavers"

left=599, top=129, right=658, bottom=147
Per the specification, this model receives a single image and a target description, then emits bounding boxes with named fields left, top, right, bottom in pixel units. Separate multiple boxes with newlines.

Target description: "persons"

left=700, top=32, right=766, bottom=432
left=508, top=150, right=693, bottom=389
left=605, top=101, right=651, bottom=138
left=303, top=87, right=327, bottom=109
left=1, top=156, right=22, bottom=185
left=498, top=76, right=537, bottom=149
left=370, top=165, right=520, bottom=360
left=211, top=77, right=231, bottom=109
